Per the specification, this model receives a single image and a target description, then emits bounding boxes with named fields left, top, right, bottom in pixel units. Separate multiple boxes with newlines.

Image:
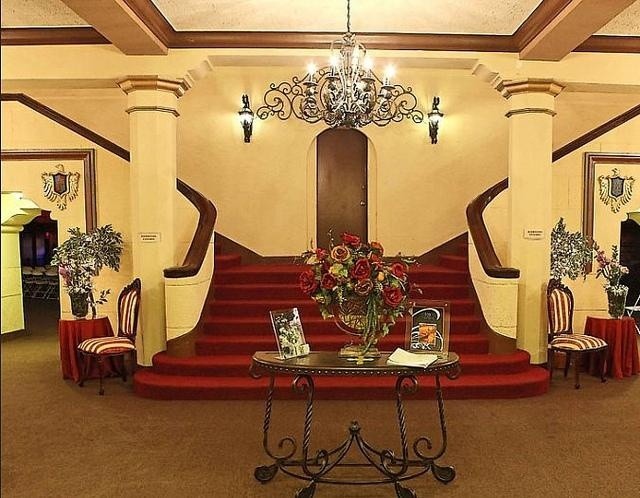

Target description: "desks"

left=57, top=314, right=122, bottom=383
left=583, top=317, right=639, bottom=382
left=250, top=349, right=464, bottom=498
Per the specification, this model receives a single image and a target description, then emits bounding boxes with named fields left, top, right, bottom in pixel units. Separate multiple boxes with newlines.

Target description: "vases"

left=601, top=284, right=626, bottom=317
left=327, top=301, right=383, bottom=362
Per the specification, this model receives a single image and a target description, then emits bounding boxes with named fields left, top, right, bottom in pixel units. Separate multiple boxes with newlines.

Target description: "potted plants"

left=50, top=224, right=125, bottom=319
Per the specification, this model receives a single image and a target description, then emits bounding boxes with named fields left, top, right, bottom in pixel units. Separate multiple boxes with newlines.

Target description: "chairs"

left=76, top=278, right=143, bottom=396
left=625, top=295, right=640, bottom=334
left=545, top=279, right=610, bottom=389
left=22, top=264, right=59, bottom=301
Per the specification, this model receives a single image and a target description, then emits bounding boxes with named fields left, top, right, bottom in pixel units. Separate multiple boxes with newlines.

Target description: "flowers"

left=590, top=246, right=631, bottom=289
left=295, top=228, right=420, bottom=331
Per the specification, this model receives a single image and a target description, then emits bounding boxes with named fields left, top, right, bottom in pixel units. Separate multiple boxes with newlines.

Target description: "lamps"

left=235, top=92, right=254, bottom=144
left=428, top=94, right=444, bottom=146
left=256, top=0, right=422, bottom=132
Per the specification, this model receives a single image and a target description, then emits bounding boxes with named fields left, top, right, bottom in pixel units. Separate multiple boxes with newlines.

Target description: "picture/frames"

left=270, top=307, right=311, bottom=360
left=403, top=298, right=451, bottom=356
